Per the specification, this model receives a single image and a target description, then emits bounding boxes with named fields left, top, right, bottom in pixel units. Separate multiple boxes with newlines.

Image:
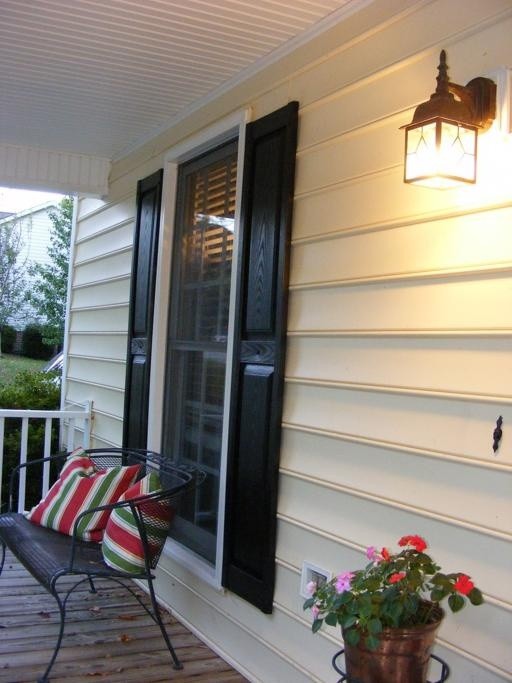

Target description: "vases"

left=338, top=602, right=445, bottom=683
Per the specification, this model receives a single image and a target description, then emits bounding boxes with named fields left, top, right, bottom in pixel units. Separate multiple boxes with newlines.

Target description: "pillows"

left=23, top=448, right=141, bottom=544
left=101, top=473, right=172, bottom=575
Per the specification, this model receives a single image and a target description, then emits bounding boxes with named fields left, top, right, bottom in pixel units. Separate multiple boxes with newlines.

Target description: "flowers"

left=301, top=535, right=483, bottom=651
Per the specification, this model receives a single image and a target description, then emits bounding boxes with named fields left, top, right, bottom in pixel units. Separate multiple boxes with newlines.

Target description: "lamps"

left=403, top=49, right=498, bottom=185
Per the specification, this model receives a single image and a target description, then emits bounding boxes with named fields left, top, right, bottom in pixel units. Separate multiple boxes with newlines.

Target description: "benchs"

left=1, top=446, right=209, bottom=680
